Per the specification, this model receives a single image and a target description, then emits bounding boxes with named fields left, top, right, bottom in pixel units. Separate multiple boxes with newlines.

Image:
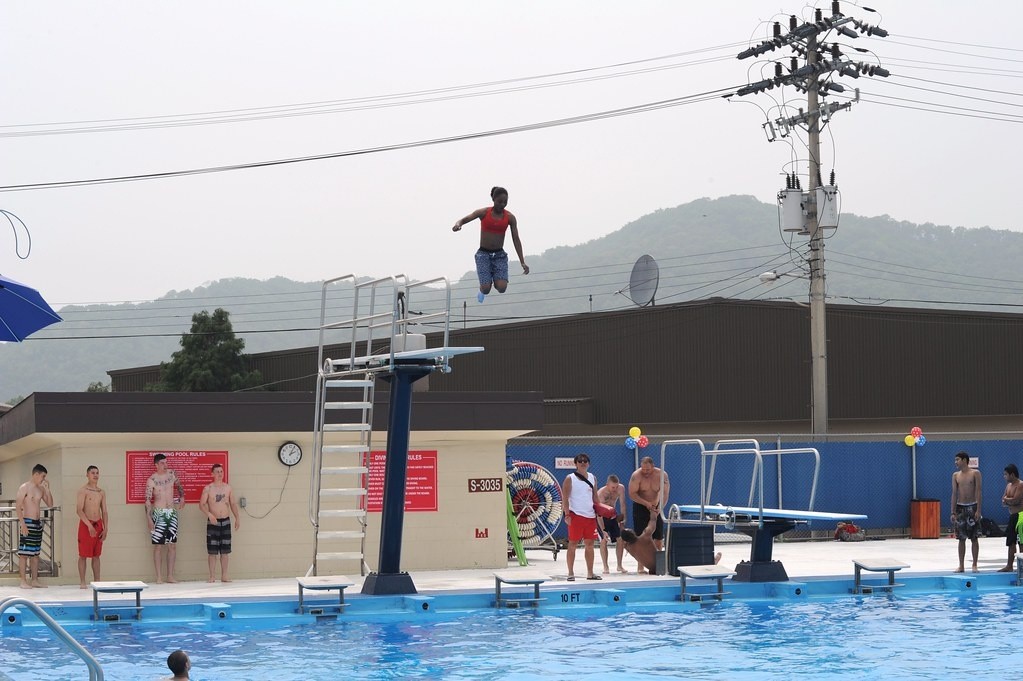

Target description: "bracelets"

left=950, top=512, right=957, bottom=516
left=564, top=514, right=570, bottom=517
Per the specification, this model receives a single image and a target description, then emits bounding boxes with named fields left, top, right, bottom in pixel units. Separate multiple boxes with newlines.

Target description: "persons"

left=561, top=453, right=603, bottom=582
left=949, top=451, right=983, bottom=573
left=145, top=453, right=186, bottom=585
left=451, top=186, right=530, bottom=304
left=75, top=464, right=109, bottom=589
left=597, top=474, right=628, bottom=574
left=615, top=456, right=722, bottom=576
left=16, top=463, right=54, bottom=589
left=198, top=463, right=241, bottom=584
left=996, top=463, right=1023, bottom=572
left=152, top=649, right=195, bottom=681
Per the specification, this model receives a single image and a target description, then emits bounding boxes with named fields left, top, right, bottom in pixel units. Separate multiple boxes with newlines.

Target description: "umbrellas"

left=0, top=273, right=65, bottom=344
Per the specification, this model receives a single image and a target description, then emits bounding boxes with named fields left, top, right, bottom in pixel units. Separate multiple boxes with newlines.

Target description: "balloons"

left=624, top=426, right=649, bottom=450
left=904, top=426, right=927, bottom=447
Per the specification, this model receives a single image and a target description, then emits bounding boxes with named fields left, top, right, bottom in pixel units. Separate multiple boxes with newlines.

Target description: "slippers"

left=567, top=576, right=575, bottom=581
left=587, top=574, right=603, bottom=580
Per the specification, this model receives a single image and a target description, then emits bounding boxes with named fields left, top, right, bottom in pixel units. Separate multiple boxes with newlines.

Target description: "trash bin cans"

left=912, top=497, right=941, bottom=538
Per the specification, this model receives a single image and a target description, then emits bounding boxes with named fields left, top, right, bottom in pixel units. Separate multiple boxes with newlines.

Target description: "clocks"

left=278, top=441, right=303, bottom=466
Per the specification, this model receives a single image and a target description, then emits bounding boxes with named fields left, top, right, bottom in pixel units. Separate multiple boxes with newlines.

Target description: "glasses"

left=576, top=459, right=589, bottom=463
